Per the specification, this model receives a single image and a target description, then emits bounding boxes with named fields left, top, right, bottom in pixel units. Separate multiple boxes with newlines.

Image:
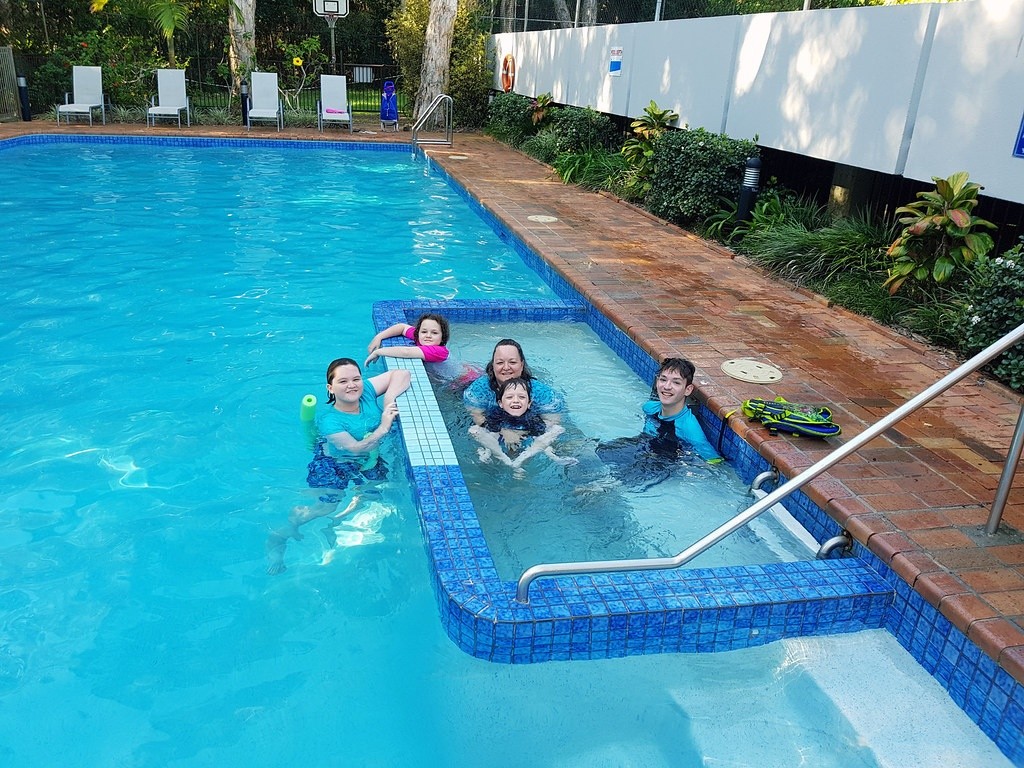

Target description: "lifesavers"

left=501, top=54, right=516, bottom=93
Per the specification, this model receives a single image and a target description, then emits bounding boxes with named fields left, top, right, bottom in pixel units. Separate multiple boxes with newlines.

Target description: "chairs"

left=146, top=69, right=191, bottom=130
left=317, top=74, right=353, bottom=135
left=246, top=72, right=284, bottom=133
left=55, top=65, right=106, bottom=128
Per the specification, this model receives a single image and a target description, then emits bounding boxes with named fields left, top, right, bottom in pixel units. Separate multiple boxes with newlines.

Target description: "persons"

left=463, top=338, right=564, bottom=454
left=467, top=377, right=579, bottom=482
left=565, top=357, right=725, bottom=495
left=364, top=313, right=486, bottom=390
left=265, top=358, right=411, bottom=575
